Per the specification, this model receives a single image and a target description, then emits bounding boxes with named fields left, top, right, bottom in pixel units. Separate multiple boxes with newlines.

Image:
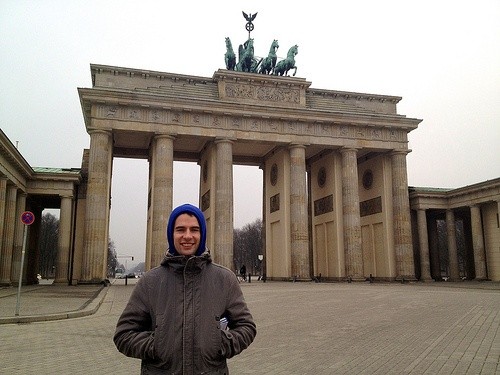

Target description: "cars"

left=126, top=272, right=135, bottom=278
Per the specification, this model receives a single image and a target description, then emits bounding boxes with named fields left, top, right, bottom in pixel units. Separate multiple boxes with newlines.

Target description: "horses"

left=224, top=37, right=238, bottom=71
left=258, top=38, right=279, bottom=75
left=237, top=38, right=254, bottom=72
left=271, top=45, right=298, bottom=76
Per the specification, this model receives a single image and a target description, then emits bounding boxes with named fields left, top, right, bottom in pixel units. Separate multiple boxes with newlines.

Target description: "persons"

left=240, top=263, right=246, bottom=280
left=111, top=204, right=258, bottom=375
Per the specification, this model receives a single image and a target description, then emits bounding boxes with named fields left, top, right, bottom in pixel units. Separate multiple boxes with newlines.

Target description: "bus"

left=115, top=268, right=124, bottom=279
